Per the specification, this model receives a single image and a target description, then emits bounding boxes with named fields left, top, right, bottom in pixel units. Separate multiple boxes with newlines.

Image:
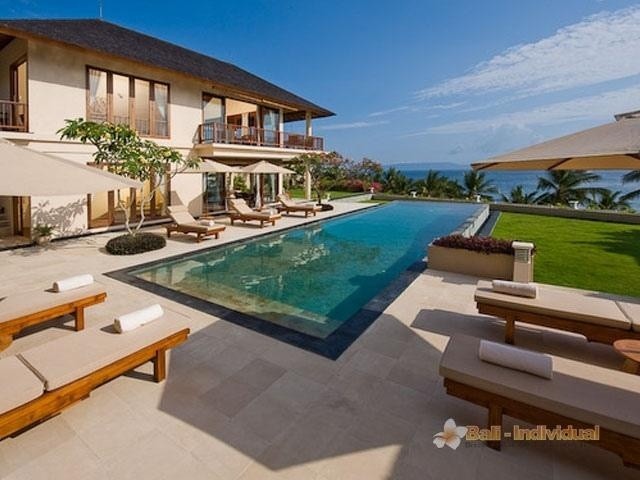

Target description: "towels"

left=51, top=273, right=94, bottom=294
left=479, top=339, right=553, bottom=381
left=113, top=304, right=165, bottom=334
left=491, top=279, right=536, bottom=300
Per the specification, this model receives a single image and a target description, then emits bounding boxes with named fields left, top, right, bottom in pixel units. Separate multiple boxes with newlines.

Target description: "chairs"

left=0, top=309, right=191, bottom=445
left=242, top=135, right=314, bottom=150
left=439, top=332, right=640, bottom=471
left=162, top=192, right=323, bottom=244
left=0, top=279, right=107, bottom=353
left=473, top=277, right=640, bottom=344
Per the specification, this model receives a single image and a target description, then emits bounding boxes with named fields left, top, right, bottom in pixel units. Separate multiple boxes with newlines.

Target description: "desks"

left=613, top=339, right=640, bottom=376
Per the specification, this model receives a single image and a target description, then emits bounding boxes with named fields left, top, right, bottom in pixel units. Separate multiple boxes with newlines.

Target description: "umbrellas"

left=469, top=110, right=640, bottom=171
left=0, top=137, right=143, bottom=197
left=165, top=159, right=234, bottom=216
left=233, top=160, right=297, bottom=208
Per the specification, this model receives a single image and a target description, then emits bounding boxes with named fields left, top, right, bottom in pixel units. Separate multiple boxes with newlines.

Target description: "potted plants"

left=34, top=222, right=57, bottom=246
left=427, top=232, right=535, bottom=282
